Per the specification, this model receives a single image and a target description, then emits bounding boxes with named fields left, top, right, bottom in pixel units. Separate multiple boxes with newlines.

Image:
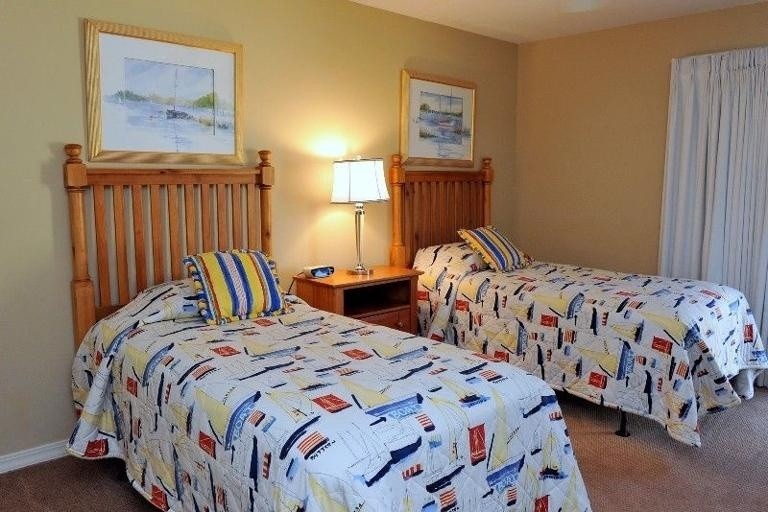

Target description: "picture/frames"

left=399, top=67, right=478, bottom=169
left=83, top=20, right=246, bottom=167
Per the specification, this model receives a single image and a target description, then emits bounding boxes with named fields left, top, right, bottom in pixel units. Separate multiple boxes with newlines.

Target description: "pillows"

left=456, top=224, right=533, bottom=274
left=185, top=250, right=292, bottom=325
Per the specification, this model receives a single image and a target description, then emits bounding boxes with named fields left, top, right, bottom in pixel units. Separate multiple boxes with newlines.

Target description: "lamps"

left=328, top=156, right=390, bottom=277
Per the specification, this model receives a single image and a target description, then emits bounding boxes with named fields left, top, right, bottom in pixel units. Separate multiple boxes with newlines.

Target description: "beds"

left=391, top=152, right=767, bottom=452
left=61, top=143, right=592, bottom=512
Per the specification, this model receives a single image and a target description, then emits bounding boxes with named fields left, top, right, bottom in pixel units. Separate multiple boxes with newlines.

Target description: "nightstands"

left=292, top=264, right=424, bottom=336
